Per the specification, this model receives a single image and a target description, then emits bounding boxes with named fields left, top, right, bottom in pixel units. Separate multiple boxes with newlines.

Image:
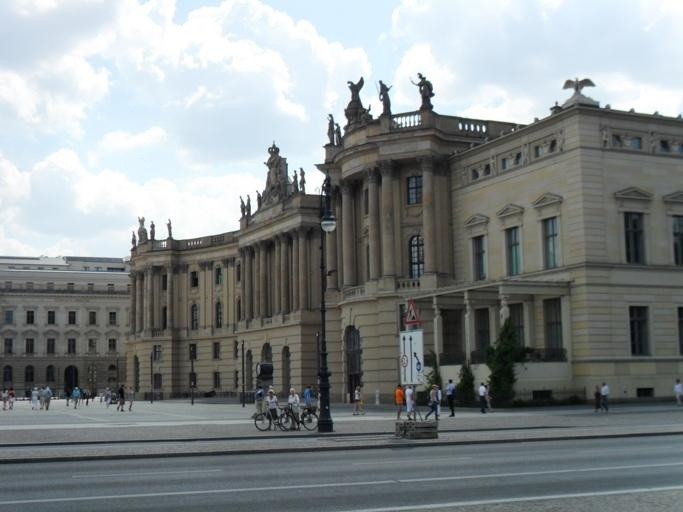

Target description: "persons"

left=351, top=385, right=365, bottom=417
left=672, top=378, right=682, bottom=406
left=411, top=73, right=435, bottom=109
left=333, top=123, right=342, bottom=146
left=149, top=221, right=154, bottom=238
left=245, top=195, right=251, bottom=215
left=393, top=379, right=455, bottom=421
left=239, top=196, right=245, bottom=217
left=0, top=381, right=136, bottom=412
left=255, top=189, right=260, bottom=209
left=166, top=218, right=172, bottom=236
left=600, top=382, right=610, bottom=412
left=291, top=170, right=297, bottom=192
left=592, top=384, right=602, bottom=411
left=249, top=385, right=314, bottom=433
left=483, top=385, right=492, bottom=413
left=298, top=168, right=306, bottom=193
left=477, top=382, right=487, bottom=413
left=327, top=113, right=335, bottom=146
left=377, top=80, right=393, bottom=116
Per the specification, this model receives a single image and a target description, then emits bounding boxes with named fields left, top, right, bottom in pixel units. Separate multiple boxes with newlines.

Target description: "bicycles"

left=254, top=402, right=319, bottom=431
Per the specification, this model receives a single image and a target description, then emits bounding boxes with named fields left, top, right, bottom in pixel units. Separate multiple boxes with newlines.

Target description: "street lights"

left=315, top=176, right=337, bottom=431
left=241, top=340, right=244, bottom=406
left=190, top=345, right=194, bottom=404
